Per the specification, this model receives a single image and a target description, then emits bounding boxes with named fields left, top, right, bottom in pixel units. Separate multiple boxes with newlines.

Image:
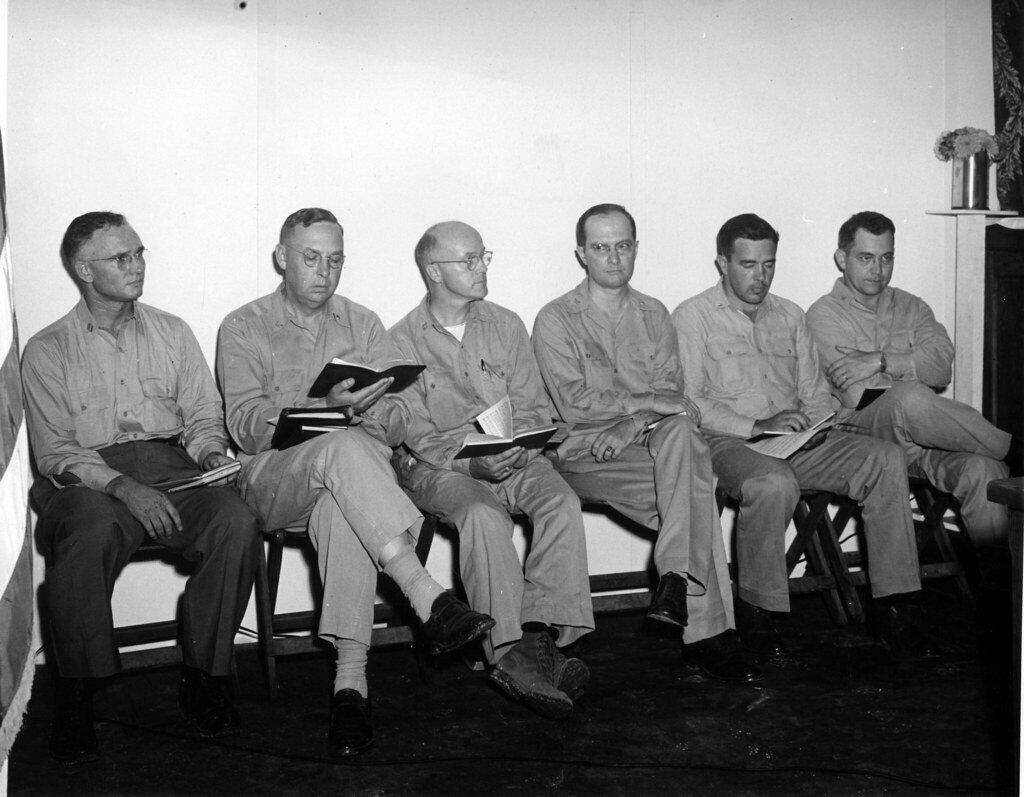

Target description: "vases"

left=951, top=148, right=990, bottom=211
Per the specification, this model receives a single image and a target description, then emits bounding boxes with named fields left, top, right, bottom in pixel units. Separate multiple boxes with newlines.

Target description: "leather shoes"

left=681, top=637, right=765, bottom=684
left=418, top=592, right=495, bottom=654
left=329, top=688, right=374, bottom=756
left=738, top=607, right=790, bottom=656
left=647, top=571, right=688, bottom=629
left=882, top=599, right=943, bottom=658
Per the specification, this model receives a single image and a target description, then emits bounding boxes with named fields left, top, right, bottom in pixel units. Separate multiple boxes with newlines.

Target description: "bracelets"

left=879, top=350, right=887, bottom=373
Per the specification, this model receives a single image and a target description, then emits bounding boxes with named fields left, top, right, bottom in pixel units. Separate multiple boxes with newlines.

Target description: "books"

left=270, top=405, right=353, bottom=451
left=744, top=412, right=836, bottom=443
left=454, top=395, right=558, bottom=460
left=153, top=460, right=242, bottom=493
left=307, top=358, right=427, bottom=399
left=856, top=385, right=946, bottom=411
left=643, top=411, right=687, bottom=434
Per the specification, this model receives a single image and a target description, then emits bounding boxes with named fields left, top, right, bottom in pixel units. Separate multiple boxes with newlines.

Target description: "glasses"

left=285, top=242, right=345, bottom=268
left=429, top=251, right=493, bottom=271
left=83, top=249, right=150, bottom=271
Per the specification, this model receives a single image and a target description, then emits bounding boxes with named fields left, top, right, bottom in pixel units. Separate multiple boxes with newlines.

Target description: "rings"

left=842, top=374, right=848, bottom=382
left=506, top=467, right=510, bottom=471
left=607, top=446, right=613, bottom=453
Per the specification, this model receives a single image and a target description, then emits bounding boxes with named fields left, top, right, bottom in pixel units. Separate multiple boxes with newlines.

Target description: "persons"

left=388, top=221, right=596, bottom=719
left=808, top=215, right=1023, bottom=623
left=674, top=213, right=944, bottom=663
left=215, top=207, right=495, bottom=756
left=20, top=210, right=259, bottom=741
left=532, top=204, right=802, bottom=683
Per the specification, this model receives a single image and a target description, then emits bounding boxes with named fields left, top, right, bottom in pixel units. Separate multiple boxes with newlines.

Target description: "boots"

left=490, top=631, right=573, bottom=719
left=551, top=645, right=590, bottom=704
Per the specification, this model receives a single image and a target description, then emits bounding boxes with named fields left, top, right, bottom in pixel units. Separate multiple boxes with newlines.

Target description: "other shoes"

left=47, top=705, right=95, bottom=765
left=175, top=677, right=243, bottom=738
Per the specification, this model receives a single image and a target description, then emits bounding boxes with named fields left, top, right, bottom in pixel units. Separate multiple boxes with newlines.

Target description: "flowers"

left=933, top=126, right=1007, bottom=163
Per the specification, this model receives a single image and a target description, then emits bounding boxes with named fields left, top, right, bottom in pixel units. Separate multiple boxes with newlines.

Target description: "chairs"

left=114, top=474, right=968, bottom=684
